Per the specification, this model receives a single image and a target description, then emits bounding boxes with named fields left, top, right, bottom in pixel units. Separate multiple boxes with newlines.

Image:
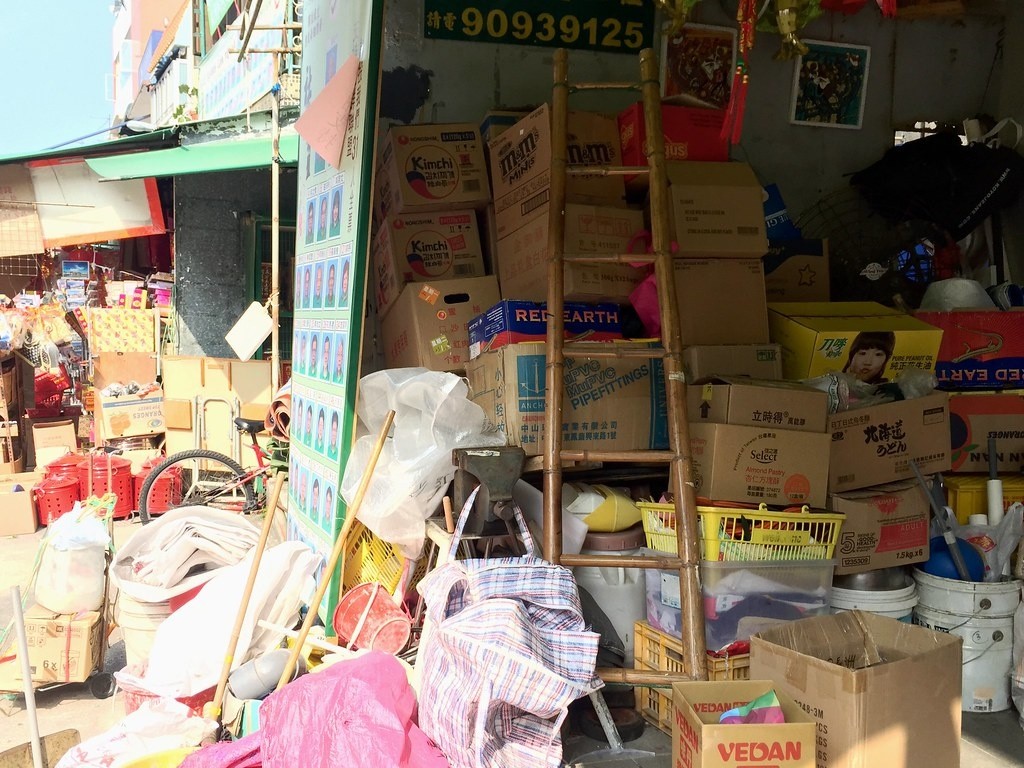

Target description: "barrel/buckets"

left=829, top=575, right=920, bottom=624
left=117, top=588, right=172, bottom=666
left=333, top=580, right=411, bottom=657
left=911, top=564, right=1024, bottom=712
left=573, top=525, right=646, bottom=693
left=116, top=661, right=218, bottom=719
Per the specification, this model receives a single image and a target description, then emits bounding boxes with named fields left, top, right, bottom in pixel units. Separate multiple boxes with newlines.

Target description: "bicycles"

left=138, top=417, right=272, bottom=526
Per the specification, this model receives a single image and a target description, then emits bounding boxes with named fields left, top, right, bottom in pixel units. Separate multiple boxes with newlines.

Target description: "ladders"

left=547, top=48, right=709, bottom=689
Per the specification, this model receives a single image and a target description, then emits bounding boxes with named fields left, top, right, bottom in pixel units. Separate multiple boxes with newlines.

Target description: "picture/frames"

left=788, top=38, right=871, bottom=131
left=658, top=21, right=738, bottom=109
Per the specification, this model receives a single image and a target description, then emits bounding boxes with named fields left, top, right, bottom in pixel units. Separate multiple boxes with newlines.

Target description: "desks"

left=161, top=354, right=271, bottom=504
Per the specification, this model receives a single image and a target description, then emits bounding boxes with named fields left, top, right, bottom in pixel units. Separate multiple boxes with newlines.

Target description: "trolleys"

left=35, top=446, right=118, bottom=700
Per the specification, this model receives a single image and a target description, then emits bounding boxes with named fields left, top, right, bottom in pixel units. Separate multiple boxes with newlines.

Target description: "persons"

left=841, top=331, right=906, bottom=403
left=295, top=257, right=355, bottom=311
left=289, top=393, right=343, bottom=464
left=304, top=183, right=344, bottom=245
left=287, top=456, right=334, bottom=584
left=291, top=331, right=344, bottom=386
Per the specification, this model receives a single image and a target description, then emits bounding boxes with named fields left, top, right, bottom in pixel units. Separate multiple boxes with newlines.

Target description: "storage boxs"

left=31, top=350, right=166, bottom=469
left=0, top=480, right=38, bottom=538
left=105, top=270, right=174, bottom=311
left=0, top=365, right=17, bottom=407
left=0, top=472, right=46, bottom=485
left=0, top=447, right=23, bottom=474
left=369, top=101, right=1024, bottom=768
left=221, top=683, right=264, bottom=741
left=15, top=602, right=103, bottom=682
